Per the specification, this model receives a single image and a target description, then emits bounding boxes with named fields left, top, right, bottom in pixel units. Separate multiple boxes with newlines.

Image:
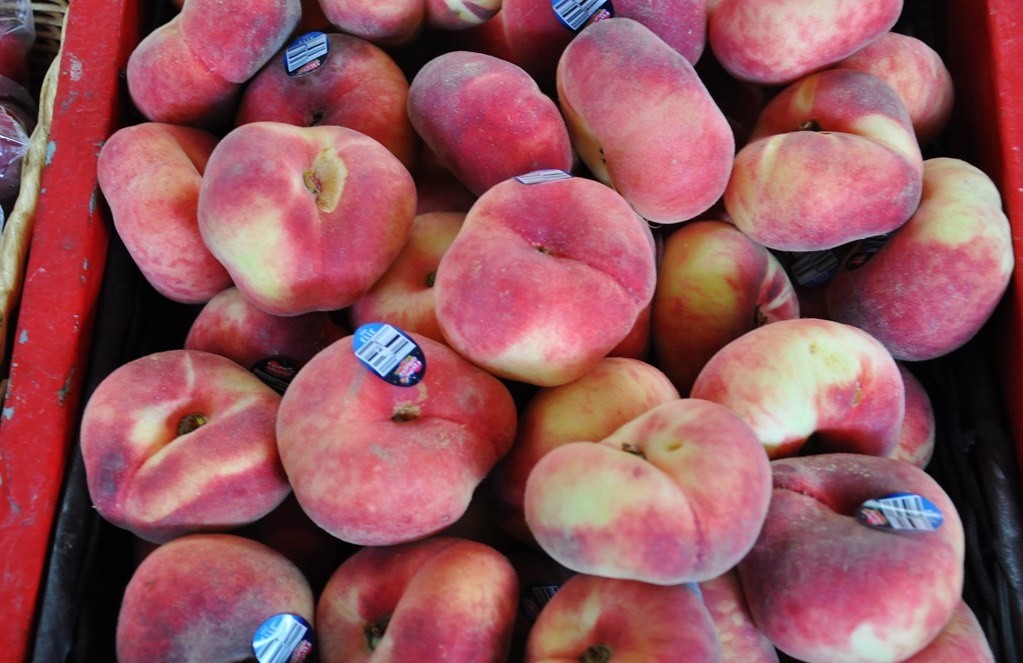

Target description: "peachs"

left=0, top=0, right=1017, bottom=663
